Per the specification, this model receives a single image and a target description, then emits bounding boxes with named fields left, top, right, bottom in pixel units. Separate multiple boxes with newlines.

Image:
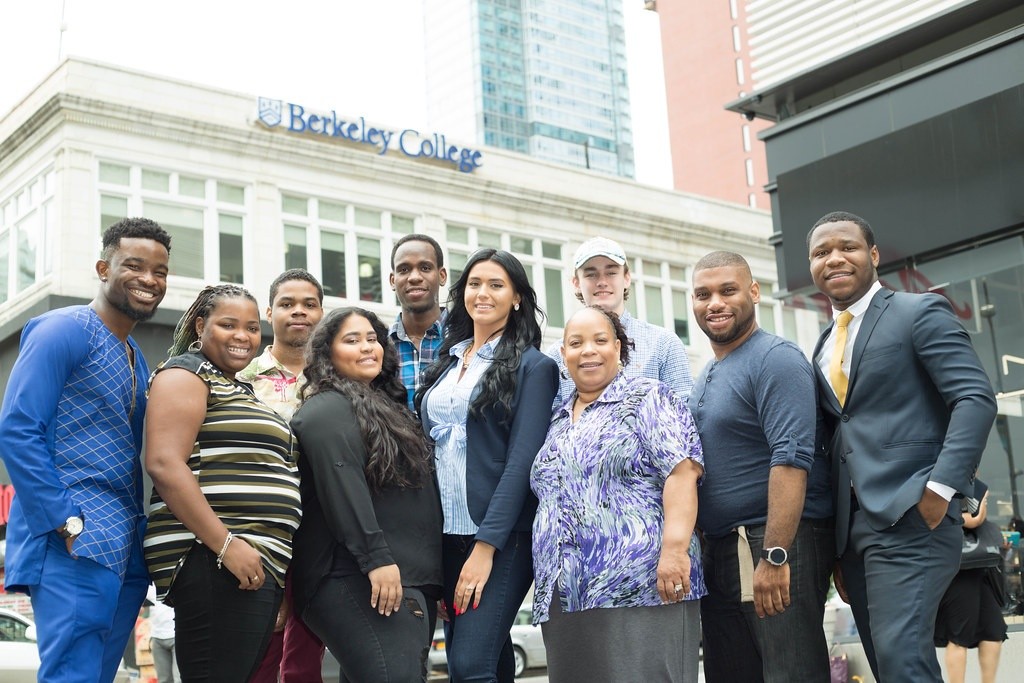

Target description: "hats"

left=574, top=236, right=627, bottom=270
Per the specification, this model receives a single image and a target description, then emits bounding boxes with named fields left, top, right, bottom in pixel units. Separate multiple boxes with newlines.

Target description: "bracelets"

left=217, top=531, right=232, bottom=569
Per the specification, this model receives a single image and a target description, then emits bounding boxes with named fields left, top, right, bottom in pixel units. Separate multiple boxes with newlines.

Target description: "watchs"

left=59, top=516, right=84, bottom=539
left=760, top=547, right=791, bottom=567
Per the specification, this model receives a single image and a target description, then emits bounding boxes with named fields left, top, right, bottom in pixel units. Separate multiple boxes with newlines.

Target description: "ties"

left=829, top=310, right=854, bottom=410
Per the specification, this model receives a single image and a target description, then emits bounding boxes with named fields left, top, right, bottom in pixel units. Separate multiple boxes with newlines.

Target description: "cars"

left=0, top=609, right=129, bottom=683
left=427, top=603, right=548, bottom=679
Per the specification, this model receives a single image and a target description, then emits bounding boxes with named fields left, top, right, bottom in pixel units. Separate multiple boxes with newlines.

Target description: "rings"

left=466, top=586, right=474, bottom=591
left=675, top=583, right=683, bottom=592
left=251, top=575, right=258, bottom=582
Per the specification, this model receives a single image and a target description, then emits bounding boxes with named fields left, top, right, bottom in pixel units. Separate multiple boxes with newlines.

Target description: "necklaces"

left=464, top=341, right=472, bottom=368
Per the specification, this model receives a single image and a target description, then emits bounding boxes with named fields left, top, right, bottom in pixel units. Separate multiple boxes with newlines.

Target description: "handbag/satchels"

left=958, top=520, right=1001, bottom=571
left=829, top=641, right=848, bottom=683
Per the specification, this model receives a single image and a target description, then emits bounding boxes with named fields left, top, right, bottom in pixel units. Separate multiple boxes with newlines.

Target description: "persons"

left=144, top=284, right=303, bottom=683
left=0, top=218, right=171, bottom=683
left=149, top=577, right=182, bottom=683
left=689, top=251, right=840, bottom=682
left=290, top=305, right=447, bottom=683
left=385, top=234, right=451, bottom=411
left=810, top=211, right=999, bottom=683
left=932, top=467, right=1009, bottom=683
left=532, top=306, right=708, bottom=683
left=413, top=247, right=560, bottom=683
left=234, top=268, right=322, bottom=683
left=545, top=236, right=693, bottom=412
